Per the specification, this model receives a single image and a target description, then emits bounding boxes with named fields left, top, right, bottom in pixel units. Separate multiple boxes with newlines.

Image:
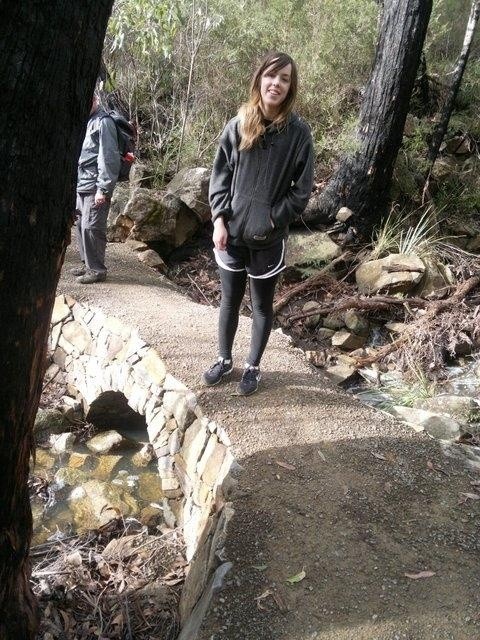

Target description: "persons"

left=70, top=91, right=121, bottom=285
left=199, top=53, right=313, bottom=398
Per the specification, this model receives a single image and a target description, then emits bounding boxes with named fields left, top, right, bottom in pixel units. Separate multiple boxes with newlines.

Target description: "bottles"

left=124, top=150, right=134, bottom=162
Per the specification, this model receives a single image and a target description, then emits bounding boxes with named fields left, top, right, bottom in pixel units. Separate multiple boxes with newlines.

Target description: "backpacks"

left=99, top=110, right=137, bottom=182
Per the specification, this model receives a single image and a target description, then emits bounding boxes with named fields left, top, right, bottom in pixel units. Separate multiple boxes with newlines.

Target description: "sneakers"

left=70, top=263, right=105, bottom=283
left=203, top=358, right=234, bottom=386
left=236, top=367, right=262, bottom=395
